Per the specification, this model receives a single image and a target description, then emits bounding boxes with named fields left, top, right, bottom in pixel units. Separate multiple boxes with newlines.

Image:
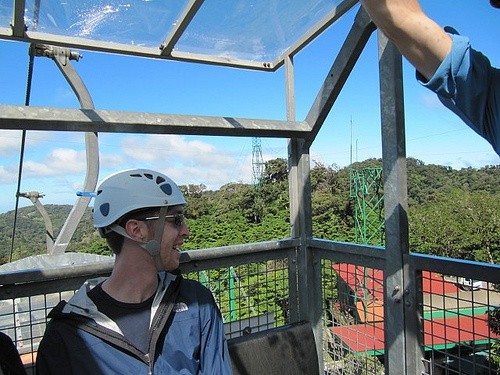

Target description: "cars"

left=450, top=276, right=482, bottom=291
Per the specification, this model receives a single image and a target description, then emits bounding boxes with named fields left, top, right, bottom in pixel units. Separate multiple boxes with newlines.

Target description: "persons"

left=33, top=170, right=237, bottom=375
left=364, top=0, right=499, bottom=157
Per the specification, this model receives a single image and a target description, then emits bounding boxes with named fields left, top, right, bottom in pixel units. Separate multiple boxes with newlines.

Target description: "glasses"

left=130, top=213, right=185, bottom=227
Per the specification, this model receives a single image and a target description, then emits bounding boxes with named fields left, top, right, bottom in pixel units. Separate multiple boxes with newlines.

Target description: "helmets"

left=92, top=168, right=186, bottom=229
